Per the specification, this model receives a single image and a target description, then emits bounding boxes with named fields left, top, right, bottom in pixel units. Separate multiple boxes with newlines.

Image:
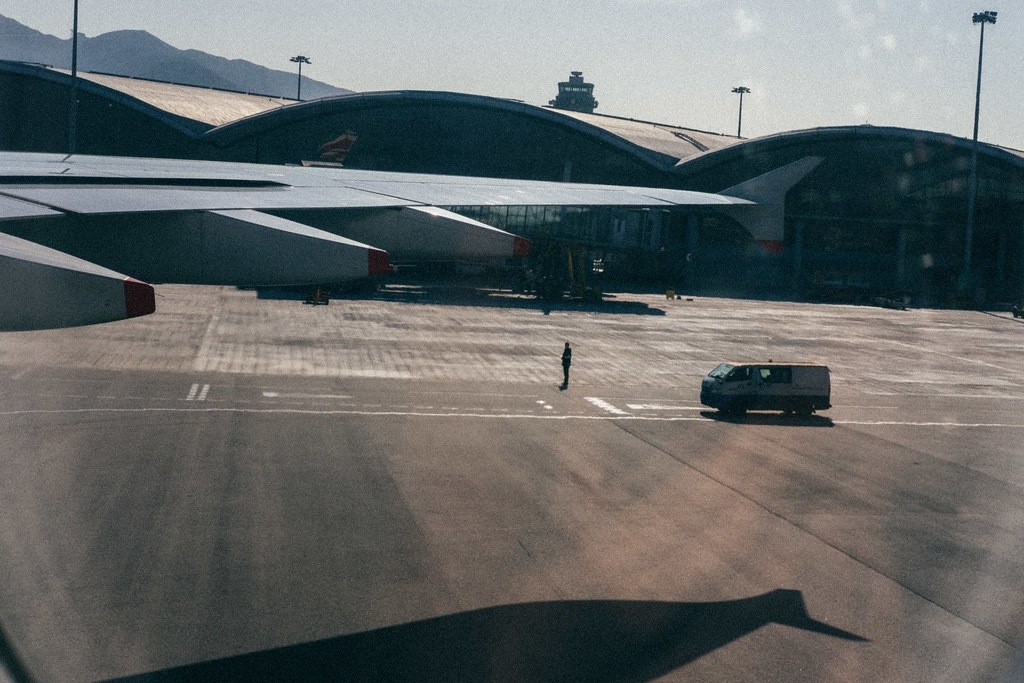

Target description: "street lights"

left=289, top=55, right=313, bottom=102
left=731, top=84, right=752, bottom=139
left=962, top=6, right=1000, bottom=267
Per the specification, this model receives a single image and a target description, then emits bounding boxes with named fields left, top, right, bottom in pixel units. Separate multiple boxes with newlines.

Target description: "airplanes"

left=0, top=149, right=758, bottom=333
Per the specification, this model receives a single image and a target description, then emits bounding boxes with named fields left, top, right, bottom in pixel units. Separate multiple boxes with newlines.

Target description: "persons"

left=558, top=342, right=572, bottom=390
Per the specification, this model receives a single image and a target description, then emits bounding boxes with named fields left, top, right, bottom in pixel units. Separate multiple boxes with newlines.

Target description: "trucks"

left=699, top=359, right=832, bottom=423
